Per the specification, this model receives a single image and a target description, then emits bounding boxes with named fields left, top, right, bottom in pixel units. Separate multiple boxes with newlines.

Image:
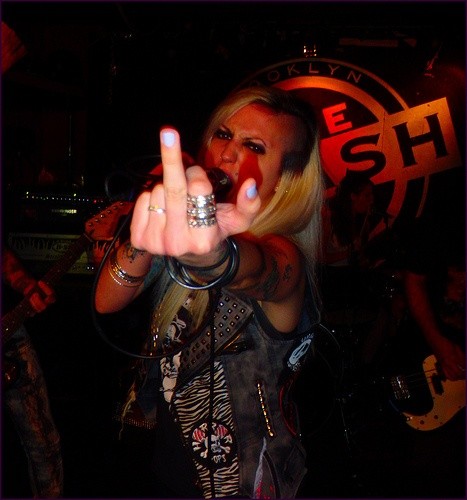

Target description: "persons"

left=324, top=172, right=467, bottom=500
left=0, top=165, right=67, bottom=500
left=96, top=84, right=329, bottom=500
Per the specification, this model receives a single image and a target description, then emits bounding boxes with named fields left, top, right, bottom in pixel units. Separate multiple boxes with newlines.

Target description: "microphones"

left=144, top=168, right=233, bottom=198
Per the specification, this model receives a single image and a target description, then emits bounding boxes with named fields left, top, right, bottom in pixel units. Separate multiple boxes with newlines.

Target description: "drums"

left=293, top=322, right=345, bottom=432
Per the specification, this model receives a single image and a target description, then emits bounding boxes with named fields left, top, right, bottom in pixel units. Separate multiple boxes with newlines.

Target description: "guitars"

left=383, top=347, right=466, bottom=431
left=0, top=199, right=134, bottom=346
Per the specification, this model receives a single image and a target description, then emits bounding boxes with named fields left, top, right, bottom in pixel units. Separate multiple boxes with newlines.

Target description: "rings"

left=150, top=204, right=164, bottom=215
left=186, top=195, right=218, bottom=227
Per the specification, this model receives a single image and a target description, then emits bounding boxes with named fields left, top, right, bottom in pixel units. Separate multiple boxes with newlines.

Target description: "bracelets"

left=167, top=238, right=239, bottom=291
left=108, top=244, right=144, bottom=288
